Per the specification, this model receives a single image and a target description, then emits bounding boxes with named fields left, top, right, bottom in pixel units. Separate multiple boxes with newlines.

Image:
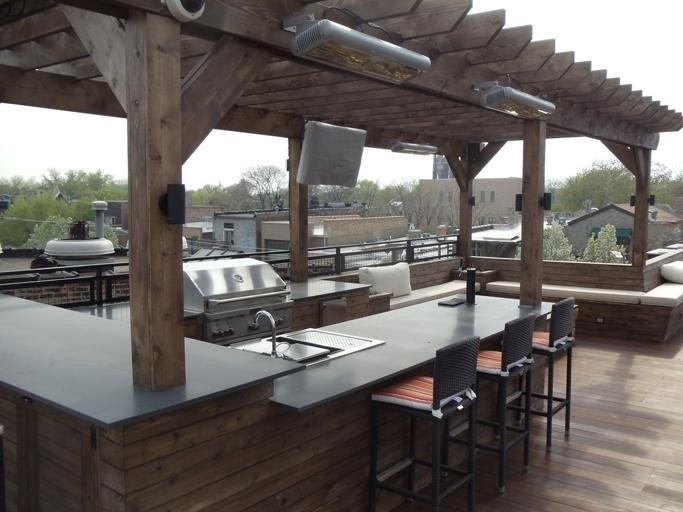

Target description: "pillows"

left=660, top=260, right=683, bottom=285
left=359, top=260, right=413, bottom=300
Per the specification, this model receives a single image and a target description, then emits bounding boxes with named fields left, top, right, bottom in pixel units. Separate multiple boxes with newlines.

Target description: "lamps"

left=291, top=17, right=433, bottom=92
left=470, top=80, right=559, bottom=125
left=389, top=140, right=441, bottom=158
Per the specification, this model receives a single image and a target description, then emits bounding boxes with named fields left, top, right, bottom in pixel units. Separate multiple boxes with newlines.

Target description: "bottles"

left=31, top=253, right=58, bottom=274
left=465, top=267, right=477, bottom=305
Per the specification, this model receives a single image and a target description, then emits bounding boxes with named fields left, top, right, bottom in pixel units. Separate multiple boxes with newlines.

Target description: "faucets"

left=457, top=257, right=467, bottom=280
left=254, top=310, right=279, bottom=358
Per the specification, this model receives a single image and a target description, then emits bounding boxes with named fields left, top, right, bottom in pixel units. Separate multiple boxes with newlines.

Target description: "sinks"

left=228, top=336, right=345, bottom=369
